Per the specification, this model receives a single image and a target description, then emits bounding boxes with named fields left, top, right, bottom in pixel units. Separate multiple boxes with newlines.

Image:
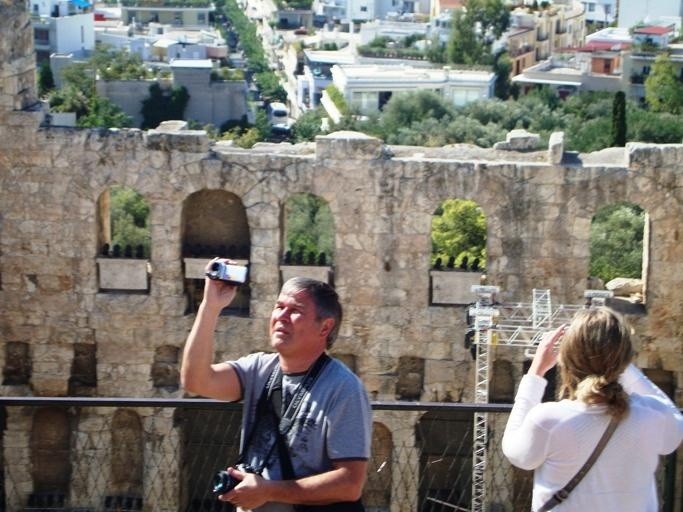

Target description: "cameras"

left=210, top=463, right=263, bottom=495
left=204, top=261, right=249, bottom=286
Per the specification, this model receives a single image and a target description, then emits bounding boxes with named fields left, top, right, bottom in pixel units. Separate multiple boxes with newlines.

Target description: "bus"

left=267, top=102, right=288, bottom=126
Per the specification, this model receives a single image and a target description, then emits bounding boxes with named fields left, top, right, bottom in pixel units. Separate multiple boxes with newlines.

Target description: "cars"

left=271, top=123, right=288, bottom=136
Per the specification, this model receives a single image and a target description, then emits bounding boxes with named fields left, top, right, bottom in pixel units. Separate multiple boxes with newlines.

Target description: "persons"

left=180, top=257, right=374, bottom=512
left=501, top=306, right=683, bottom=512
